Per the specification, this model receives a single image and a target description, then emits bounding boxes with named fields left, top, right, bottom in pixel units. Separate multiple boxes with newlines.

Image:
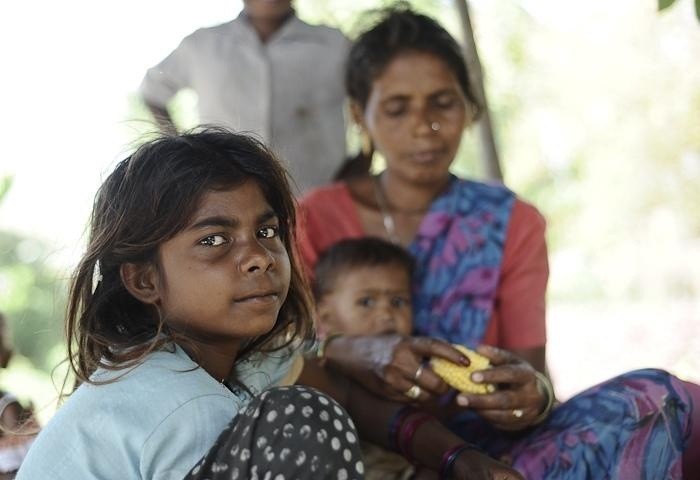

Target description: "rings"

left=403, top=383, right=422, bottom=402
left=513, top=406, right=525, bottom=419
left=412, top=363, right=427, bottom=381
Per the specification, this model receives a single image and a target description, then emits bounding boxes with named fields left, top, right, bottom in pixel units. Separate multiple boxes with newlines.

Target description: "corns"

left=428, top=343, right=499, bottom=394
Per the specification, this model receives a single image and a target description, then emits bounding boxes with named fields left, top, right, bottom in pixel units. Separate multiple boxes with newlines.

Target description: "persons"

left=307, top=232, right=420, bottom=349
left=137, top=0, right=377, bottom=217
left=0, top=312, right=42, bottom=480
left=289, top=10, right=698, bottom=479
left=12, top=123, right=520, bottom=478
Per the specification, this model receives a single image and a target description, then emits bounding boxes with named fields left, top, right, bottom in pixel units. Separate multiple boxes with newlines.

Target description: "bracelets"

left=387, top=401, right=425, bottom=452
left=315, top=325, right=344, bottom=372
left=439, top=439, right=481, bottom=477
left=533, top=366, right=558, bottom=431
left=404, top=411, right=440, bottom=469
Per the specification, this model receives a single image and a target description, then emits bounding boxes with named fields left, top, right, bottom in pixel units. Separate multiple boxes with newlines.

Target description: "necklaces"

left=372, top=181, right=425, bottom=248
